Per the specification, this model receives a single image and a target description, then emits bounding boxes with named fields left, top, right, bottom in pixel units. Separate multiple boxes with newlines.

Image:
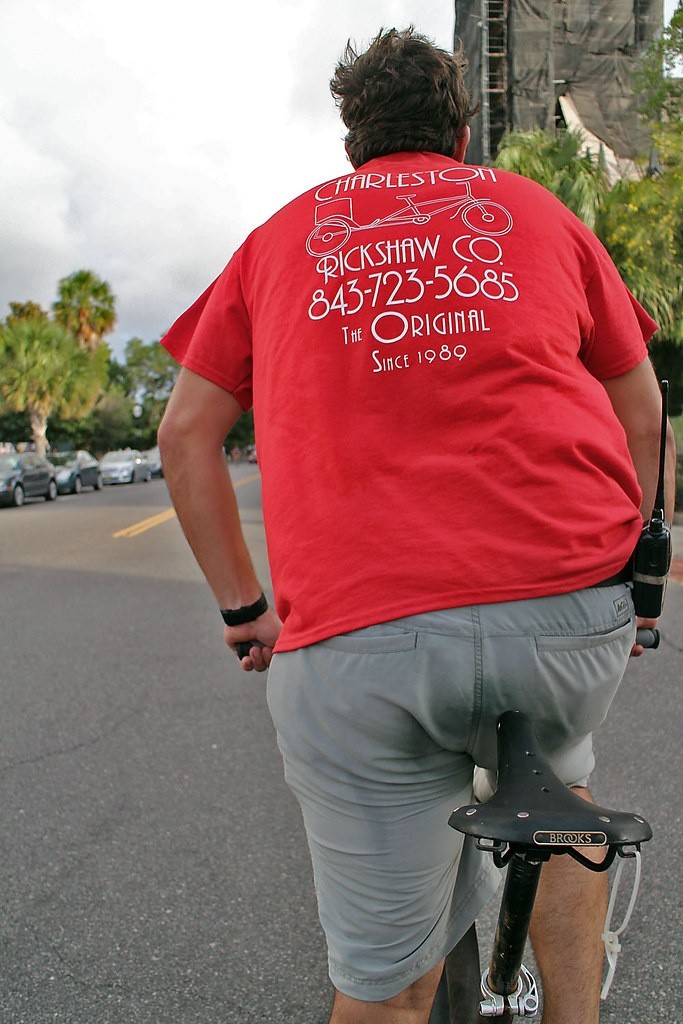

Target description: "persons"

left=247, top=443, right=255, bottom=466
left=142, top=14, right=681, bottom=1023
left=231, top=443, right=242, bottom=466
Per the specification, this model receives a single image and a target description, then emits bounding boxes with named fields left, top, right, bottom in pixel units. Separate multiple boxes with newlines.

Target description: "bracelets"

left=219, top=592, right=269, bottom=626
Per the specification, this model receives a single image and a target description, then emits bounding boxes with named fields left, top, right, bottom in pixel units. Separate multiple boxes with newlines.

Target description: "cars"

left=45, top=450, right=104, bottom=497
left=97, top=449, right=152, bottom=487
left=0, top=453, right=57, bottom=507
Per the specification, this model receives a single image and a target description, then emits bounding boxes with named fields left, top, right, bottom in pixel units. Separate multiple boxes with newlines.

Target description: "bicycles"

left=235, top=626, right=660, bottom=1024
